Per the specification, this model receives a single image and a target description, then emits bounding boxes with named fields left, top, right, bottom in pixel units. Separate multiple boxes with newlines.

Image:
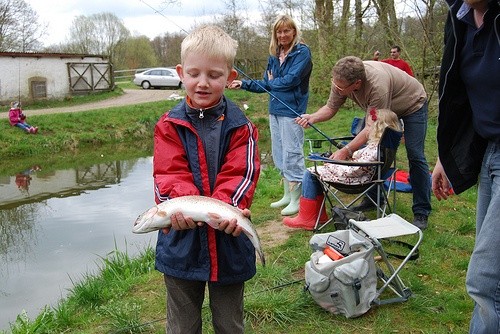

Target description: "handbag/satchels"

left=332, top=205, right=370, bottom=230
left=302, top=229, right=377, bottom=319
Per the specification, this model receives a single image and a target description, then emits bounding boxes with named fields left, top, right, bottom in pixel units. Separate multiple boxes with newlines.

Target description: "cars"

left=132, top=68, right=185, bottom=90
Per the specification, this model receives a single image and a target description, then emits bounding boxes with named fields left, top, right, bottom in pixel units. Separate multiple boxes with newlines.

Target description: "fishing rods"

left=17, top=57, right=21, bottom=117
left=139, top=0, right=344, bottom=150
left=132, top=256, right=382, bottom=328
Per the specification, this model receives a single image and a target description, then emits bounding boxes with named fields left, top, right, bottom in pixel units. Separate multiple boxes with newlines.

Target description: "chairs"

left=306, top=116, right=404, bottom=233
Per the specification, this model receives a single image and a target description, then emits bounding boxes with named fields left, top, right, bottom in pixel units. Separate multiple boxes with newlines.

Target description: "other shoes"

left=414, top=214, right=429, bottom=230
left=352, top=196, right=384, bottom=212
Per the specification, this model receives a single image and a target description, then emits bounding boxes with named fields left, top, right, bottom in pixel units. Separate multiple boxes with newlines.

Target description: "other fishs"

left=132, top=195, right=266, bottom=266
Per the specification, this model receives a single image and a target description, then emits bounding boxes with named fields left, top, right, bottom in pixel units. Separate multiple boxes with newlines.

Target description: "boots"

left=280, top=182, right=302, bottom=215
left=26, top=126, right=39, bottom=134
left=268, top=179, right=291, bottom=208
left=281, top=194, right=329, bottom=230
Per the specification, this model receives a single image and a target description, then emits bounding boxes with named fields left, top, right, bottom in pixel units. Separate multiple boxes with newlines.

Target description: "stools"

left=350, top=213, right=422, bottom=307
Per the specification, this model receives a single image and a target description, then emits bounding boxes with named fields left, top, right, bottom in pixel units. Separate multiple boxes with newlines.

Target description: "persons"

left=432, top=0, right=500, bottom=334
left=295, top=57, right=430, bottom=228
left=153, top=25, right=259, bottom=334
left=375, top=47, right=415, bottom=79
left=229, top=16, right=313, bottom=215
left=15, top=166, right=43, bottom=196
left=9, top=102, right=39, bottom=133
left=281, top=110, right=403, bottom=229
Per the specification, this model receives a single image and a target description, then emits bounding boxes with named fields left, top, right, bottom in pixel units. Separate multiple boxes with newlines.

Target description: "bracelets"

left=344, top=144, right=354, bottom=158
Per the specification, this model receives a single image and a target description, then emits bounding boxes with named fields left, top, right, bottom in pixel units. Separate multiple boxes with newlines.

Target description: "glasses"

left=330, top=78, right=355, bottom=92
